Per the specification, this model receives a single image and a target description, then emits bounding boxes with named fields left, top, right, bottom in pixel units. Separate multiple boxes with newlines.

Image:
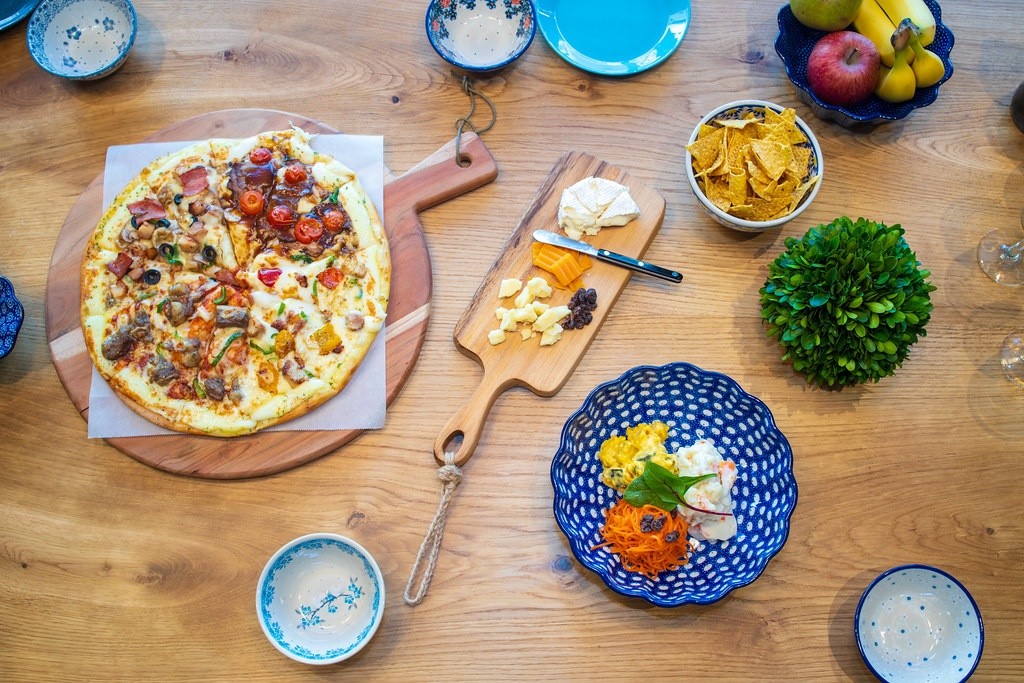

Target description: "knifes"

left=533, top=230, right=684, bottom=284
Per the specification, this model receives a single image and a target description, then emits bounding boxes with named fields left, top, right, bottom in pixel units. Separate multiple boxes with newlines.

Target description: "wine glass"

left=977, top=226, right=1024, bottom=288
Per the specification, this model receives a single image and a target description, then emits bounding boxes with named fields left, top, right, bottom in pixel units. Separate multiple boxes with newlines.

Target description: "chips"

left=685, top=106, right=820, bottom=220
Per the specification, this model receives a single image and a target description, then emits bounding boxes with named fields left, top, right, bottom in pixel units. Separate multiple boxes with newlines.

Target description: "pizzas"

left=79, top=127, right=393, bottom=437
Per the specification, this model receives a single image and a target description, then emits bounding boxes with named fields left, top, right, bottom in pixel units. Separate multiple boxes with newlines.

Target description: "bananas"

left=851, top=0, right=947, bottom=104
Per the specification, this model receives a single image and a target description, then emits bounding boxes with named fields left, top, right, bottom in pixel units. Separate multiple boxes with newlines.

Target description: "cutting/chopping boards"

left=433, top=148, right=666, bottom=467
left=46, top=107, right=499, bottom=480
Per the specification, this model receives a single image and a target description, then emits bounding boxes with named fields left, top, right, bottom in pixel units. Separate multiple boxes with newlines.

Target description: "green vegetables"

left=623, top=462, right=735, bottom=516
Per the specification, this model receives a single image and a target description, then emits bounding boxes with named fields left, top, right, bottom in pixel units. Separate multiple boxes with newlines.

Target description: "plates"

left=0, top=275, right=25, bottom=358
left=0, top=0, right=39, bottom=32
left=534, top=0, right=691, bottom=75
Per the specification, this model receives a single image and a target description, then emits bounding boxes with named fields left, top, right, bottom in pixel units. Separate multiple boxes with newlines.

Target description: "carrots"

left=590, top=499, right=696, bottom=580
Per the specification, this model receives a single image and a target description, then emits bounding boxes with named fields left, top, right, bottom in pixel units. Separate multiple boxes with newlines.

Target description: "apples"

left=808, top=31, right=881, bottom=106
left=789, top=0, right=864, bottom=32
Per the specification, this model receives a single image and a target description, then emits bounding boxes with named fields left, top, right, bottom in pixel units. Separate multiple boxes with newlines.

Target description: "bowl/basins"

left=773, top=0, right=955, bottom=121
left=425, top=0, right=536, bottom=72
left=854, top=564, right=986, bottom=683
left=256, top=532, right=386, bottom=666
left=26, top=0, right=138, bottom=81
left=685, top=99, right=823, bottom=232
left=550, top=363, right=798, bottom=605
